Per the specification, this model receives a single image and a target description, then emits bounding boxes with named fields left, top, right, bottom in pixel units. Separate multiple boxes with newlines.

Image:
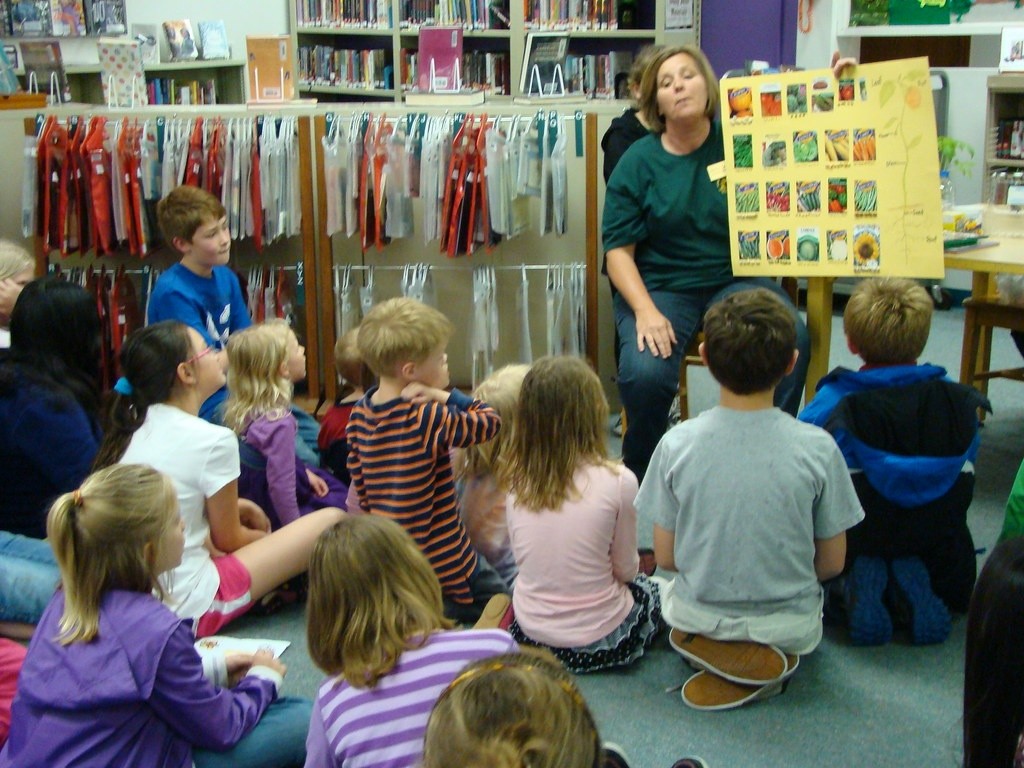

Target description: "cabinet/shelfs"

left=0, top=0, right=248, bottom=109
left=291, top=0, right=665, bottom=110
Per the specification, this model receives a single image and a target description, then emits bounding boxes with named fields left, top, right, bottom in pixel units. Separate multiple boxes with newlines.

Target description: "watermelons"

left=767, top=236, right=789, bottom=260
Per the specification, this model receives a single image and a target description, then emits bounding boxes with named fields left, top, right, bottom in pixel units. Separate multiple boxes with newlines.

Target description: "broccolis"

left=787, top=85, right=807, bottom=112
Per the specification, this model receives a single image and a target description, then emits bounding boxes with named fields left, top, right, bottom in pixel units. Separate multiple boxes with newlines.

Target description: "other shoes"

left=680, top=647, right=799, bottom=711
left=893, top=556, right=951, bottom=645
left=847, top=553, right=893, bottom=649
left=258, top=584, right=308, bottom=616
left=672, top=756, right=706, bottom=768
left=599, top=742, right=632, bottom=768
left=668, top=624, right=788, bottom=686
left=473, top=591, right=513, bottom=631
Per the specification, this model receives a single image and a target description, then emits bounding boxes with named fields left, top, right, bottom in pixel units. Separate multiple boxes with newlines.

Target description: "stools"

left=621, top=331, right=706, bottom=445
left=957, top=294, right=1024, bottom=418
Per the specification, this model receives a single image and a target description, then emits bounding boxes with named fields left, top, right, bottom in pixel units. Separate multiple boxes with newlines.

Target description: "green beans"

left=736, top=190, right=759, bottom=213
left=854, top=186, right=877, bottom=211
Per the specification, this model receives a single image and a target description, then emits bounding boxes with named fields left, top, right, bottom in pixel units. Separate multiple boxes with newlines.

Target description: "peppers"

left=827, top=183, right=848, bottom=212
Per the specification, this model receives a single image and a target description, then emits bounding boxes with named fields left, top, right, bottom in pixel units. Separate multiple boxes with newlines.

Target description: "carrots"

left=854, top=135, right=877, bottom=161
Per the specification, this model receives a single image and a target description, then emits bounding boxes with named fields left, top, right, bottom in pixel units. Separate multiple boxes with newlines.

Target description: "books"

left=0, top=0, right=634, bottom=103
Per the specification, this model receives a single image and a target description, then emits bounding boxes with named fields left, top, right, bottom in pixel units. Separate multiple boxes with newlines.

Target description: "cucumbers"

left=740, top=240, right=757, bottom=258
left=806, top=191, right=820, bottom=210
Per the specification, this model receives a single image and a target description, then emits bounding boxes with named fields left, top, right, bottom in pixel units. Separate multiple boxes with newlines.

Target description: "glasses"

left=171, top=342, right=215, bottom=386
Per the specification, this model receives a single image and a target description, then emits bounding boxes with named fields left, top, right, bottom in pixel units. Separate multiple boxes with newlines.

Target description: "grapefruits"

left=729, top=88, right=753, bottom=118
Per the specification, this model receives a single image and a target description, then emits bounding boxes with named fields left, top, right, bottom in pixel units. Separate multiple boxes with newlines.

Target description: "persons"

left=0, top=187, right=663, bottom=675
left=797, top=273, right=994, bottom=648
left=304, top=515, right=707, bottom=768
left=632, top=286, right=866, bottom=709
left=601, top=46, right=857, bottom=488
left=601, top=46, right=664, bottom=437
left=424, top=651, right=598, bottom=768
left=0, top=464, right=312, bottom=768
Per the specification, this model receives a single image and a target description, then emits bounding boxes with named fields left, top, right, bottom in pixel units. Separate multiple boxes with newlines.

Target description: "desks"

left=804, top=232, right=1024, bottom=401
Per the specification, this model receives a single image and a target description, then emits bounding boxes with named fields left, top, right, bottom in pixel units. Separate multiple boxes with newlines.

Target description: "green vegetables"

left=733, top=134, right=753, bottom=168
left=793, top=131, right=817, bottom=164
